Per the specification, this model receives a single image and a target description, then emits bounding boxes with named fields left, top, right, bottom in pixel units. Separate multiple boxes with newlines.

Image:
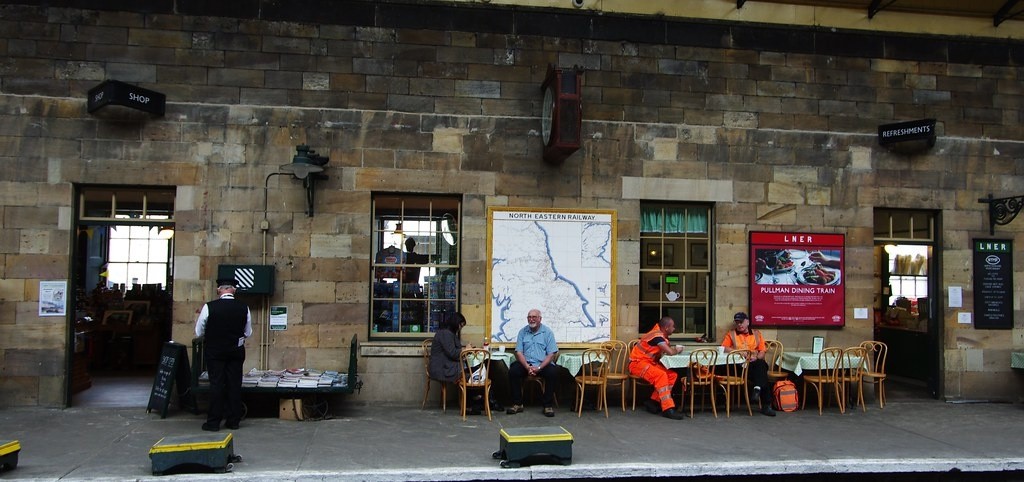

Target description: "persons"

left=195, top=278, right=253, bottom=431
left=430, top=311, right=505, bottom=412
left=809, top=252, right=840, bottom=270
left=721, top=312, right=777, bottom=417
left=506, top=309, right=558, bottom=417
left=628, top=316, right=683, bottom=420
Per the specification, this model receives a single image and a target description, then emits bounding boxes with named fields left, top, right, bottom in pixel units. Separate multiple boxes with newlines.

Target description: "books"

left=242, top=367, right=348, bottom=387
left=198, top=371, right=210, bottom=386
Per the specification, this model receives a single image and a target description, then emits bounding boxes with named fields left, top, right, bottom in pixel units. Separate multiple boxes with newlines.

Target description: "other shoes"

left=662, top=408, right=683, bottom=419
left=761, top=405, right=776, bottom=417
left=752, top=388, right=760, bottom=403
left=474, top=396, right=494, bottom=406
left=225, top=423, right=239, bottom=429
left=507, top=404, right=523, bottom=414
left=466, top=409, right=481, bottom=416
left=645, top=398, right=660, bottom=412
left=202, top=422, right=221, bottom=431
left=545, top=407, right=554, bottom=417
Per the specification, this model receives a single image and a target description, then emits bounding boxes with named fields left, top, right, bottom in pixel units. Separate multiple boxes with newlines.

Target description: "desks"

left=775, top=351, right=869, bottom=409
left=660, top=351, right=747, bottom=409
left=557, top=353, right=608, bottom=411
left=464, top=352, right=517, bottom=406
left=1010, top=352, right=1024, bottom=369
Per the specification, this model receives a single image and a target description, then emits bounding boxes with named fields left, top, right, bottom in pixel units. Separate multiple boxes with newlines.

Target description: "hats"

left=734, top=312, right=748, bottom=322
left=216, top=277, right=239, bottom=288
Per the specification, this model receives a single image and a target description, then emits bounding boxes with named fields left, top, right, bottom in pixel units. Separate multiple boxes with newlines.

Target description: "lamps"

left=280, top=143, right=331, bottom=216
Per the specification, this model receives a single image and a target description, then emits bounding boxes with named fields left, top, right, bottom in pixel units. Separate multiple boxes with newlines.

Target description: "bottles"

left=483, top=336, right=490, bottom=352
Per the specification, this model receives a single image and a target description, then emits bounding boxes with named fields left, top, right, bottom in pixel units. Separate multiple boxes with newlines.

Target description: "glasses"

left=527, top=315, right=540, bottom=319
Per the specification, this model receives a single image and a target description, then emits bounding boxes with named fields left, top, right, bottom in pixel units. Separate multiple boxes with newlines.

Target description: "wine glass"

left=764, top=250, right=780, bottom=284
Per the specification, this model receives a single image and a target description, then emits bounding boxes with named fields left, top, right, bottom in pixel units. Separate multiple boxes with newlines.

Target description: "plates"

left=797, top=267, right=839, bottom=286
left=755, top=271, right=764, bottom=284
left=788, top=249, right=806, bottom=259
left=774, top=260, right=795, bottom=272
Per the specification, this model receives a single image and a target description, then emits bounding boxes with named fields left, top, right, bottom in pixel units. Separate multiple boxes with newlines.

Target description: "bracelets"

left=538, top=366, right=541, bottom=370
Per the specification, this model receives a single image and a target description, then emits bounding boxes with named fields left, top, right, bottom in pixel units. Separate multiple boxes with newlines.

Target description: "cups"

left=676, top=344, right=684, bottom=353
left=498, top=345, right=505, bottom=353
left=718, top=346, right=725, bottom=355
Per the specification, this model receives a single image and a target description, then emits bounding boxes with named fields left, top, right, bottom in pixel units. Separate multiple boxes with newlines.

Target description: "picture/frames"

left=669, top=271, right=697, bottom=298
left=102, top=300, right=150, bottom=326
left=690, top=242, right=708, bottom=265
left=646, top=242, right=674, bottom=265
left=645, top=276, right=661, bottom=293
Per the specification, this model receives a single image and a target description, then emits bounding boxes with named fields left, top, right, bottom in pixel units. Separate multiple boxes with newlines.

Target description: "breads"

left=893, top=254, right=925, bottom=276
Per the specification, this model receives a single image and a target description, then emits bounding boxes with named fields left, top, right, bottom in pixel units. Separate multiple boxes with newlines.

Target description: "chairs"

left=759, top=340, right=788, bottom=410
left=853, top=340, right=888, bottom=409
left=459, top=349, right=493, bottom=422
left=711, top=349, right=753, bottom=418
left=801, top=347, right=843, bottom=415
left=682, top=349, right=718, bottom=419
left=826, top=347, right=867, bottom=413
left=522, top=352, right=560, bottom=407
left=422, top=338, right=463, bottom=413
left=574, top=339, right=655, bottom=418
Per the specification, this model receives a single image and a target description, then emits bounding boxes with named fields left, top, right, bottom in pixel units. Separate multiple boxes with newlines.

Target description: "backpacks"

left=772, top=379, right=799, bottom=412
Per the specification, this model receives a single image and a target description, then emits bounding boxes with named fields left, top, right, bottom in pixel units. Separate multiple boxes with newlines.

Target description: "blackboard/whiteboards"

left=147, top=345, right=191, bottom=410
